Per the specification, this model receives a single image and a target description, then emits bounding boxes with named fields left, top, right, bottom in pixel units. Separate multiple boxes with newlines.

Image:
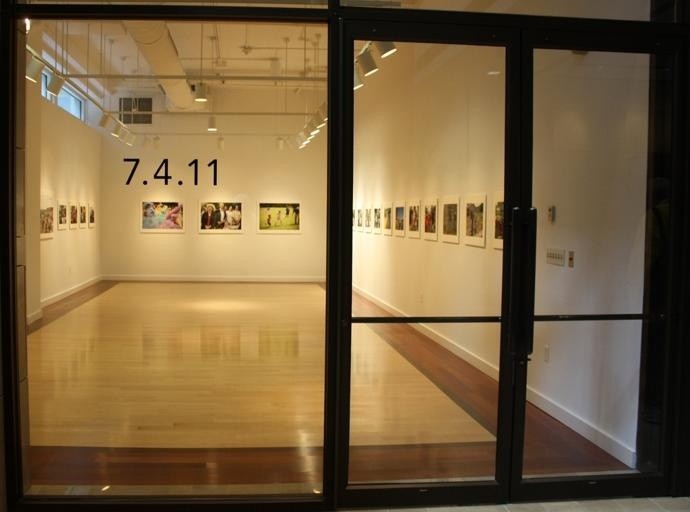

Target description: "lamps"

left=276, top=32, right=397, bottom=152
left=26, top=19, right=138, bottom=148
left=192, top=23, right=226, bottom=151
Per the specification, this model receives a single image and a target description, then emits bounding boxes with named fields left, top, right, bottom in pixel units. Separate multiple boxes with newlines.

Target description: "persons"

left=201, top=203, right=241, bottom=229
left=265, top=205, right=300, bottom=227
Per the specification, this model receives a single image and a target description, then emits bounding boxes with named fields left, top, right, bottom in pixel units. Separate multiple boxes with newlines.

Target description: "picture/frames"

left=138, top=199, right=302, bottom=234
left=39, top=196, right=95, bottom=241
left=353, top=194, right=503, bottom=250
